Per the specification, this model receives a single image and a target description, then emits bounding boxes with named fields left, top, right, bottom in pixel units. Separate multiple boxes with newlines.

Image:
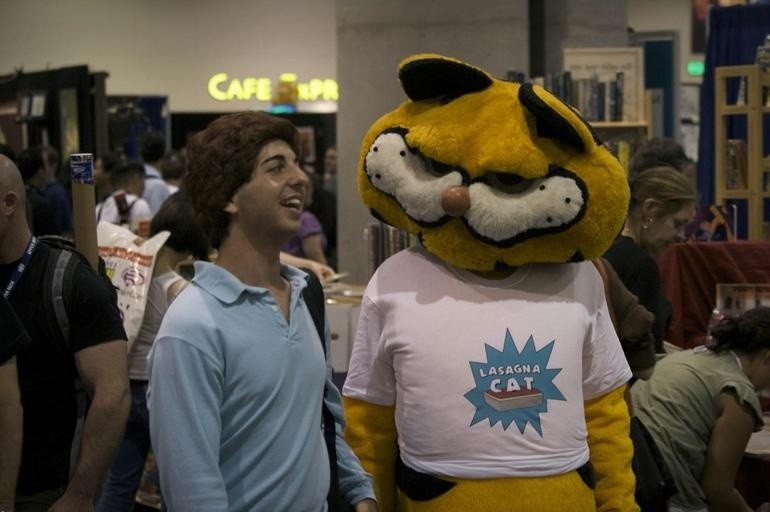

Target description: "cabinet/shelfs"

left=713, top=64, right=769, bottom=241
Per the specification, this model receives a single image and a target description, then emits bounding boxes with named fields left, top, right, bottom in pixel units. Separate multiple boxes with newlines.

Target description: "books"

left=530, top=71, right=624, bottom=123
left=602, top=141, right=632, bottom=177
left=370, top=222, right=409, bottom=273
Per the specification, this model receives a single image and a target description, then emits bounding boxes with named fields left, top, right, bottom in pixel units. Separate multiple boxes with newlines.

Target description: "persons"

left=2, top=75, right=379, bottom=512
left=596, top=167, right=769, bottom=512
left=635, top=137, right=693, bottom=181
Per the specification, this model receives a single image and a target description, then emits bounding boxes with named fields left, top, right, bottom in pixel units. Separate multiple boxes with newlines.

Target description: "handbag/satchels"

left=631, top=415, right=681, bottom=507
left=326, top=492, right=357, bottom=512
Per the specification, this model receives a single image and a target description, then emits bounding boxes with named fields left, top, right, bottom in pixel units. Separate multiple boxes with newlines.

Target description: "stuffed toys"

left=339, top=55, right=640, bottom=512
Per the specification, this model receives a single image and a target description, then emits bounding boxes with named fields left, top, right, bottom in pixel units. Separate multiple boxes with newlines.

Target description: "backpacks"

left=39, top=235, right=123, bottom=389
